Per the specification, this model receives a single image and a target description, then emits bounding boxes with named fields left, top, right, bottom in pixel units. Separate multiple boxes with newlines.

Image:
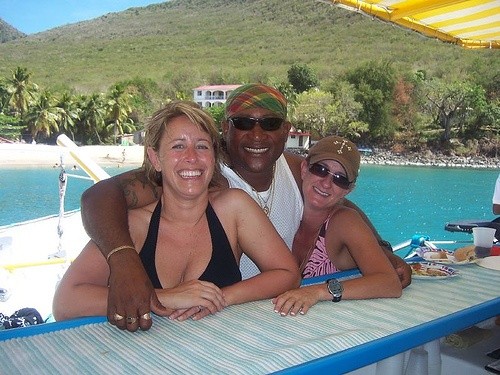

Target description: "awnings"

left=321, top=0, right=500, bottom=50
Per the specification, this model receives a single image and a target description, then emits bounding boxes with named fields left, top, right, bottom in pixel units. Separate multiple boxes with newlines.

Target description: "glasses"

left=309, top=163, right=349, bottom=189
left=227, top=116, right=283, bottom=131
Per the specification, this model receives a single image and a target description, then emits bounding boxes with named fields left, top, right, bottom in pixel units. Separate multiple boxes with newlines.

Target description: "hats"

left=308, top=136, right=361, bottom=183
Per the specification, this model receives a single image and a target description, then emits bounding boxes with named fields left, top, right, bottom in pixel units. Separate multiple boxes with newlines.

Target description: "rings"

left=126, top=317, right=139, bottom=324
left=198, top=306, right=201, bottom=312
left=114, top=313, right=125, bottom=320
left=141, top=313, right=151, bottom=320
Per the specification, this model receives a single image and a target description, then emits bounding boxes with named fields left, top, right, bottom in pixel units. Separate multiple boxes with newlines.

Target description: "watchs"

left=327, top=278, right=344, bottom=301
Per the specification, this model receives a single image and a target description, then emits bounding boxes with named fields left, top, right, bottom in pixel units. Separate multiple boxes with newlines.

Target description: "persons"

left=81, top=84, right=411, bottom=332
left=492, top=176, right=500, bottom=215
left=52, top=101, right=302, bottom=321
left=273, top=136, right=402, bottom=316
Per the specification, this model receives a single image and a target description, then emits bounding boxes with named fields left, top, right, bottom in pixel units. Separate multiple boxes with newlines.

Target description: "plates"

left=406, top=261, right=458, bottom=280
left=417, top=248, right=470, bottom=265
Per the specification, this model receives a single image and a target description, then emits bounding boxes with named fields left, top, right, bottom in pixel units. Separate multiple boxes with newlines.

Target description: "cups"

left=472, top=227, right=496, bottom=258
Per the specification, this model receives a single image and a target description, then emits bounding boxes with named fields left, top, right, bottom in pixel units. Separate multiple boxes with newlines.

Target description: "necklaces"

left=223, top=157, right=275, bottom=217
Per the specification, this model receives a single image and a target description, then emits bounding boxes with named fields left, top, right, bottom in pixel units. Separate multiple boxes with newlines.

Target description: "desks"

left=0, top=250, right=500, bottom=375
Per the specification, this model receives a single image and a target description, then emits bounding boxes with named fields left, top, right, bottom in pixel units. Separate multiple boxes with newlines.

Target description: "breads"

left=430, top=252, right=447, bottom=259
left=427, top=267, right=449, bottom=276
left=455, top=247, right=475, bottom=261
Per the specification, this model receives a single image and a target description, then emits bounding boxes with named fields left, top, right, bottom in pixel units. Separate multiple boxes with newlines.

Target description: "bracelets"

left=379, top=240, right=393, bottom=252
left=107, top=245, right=135, bottom=259
